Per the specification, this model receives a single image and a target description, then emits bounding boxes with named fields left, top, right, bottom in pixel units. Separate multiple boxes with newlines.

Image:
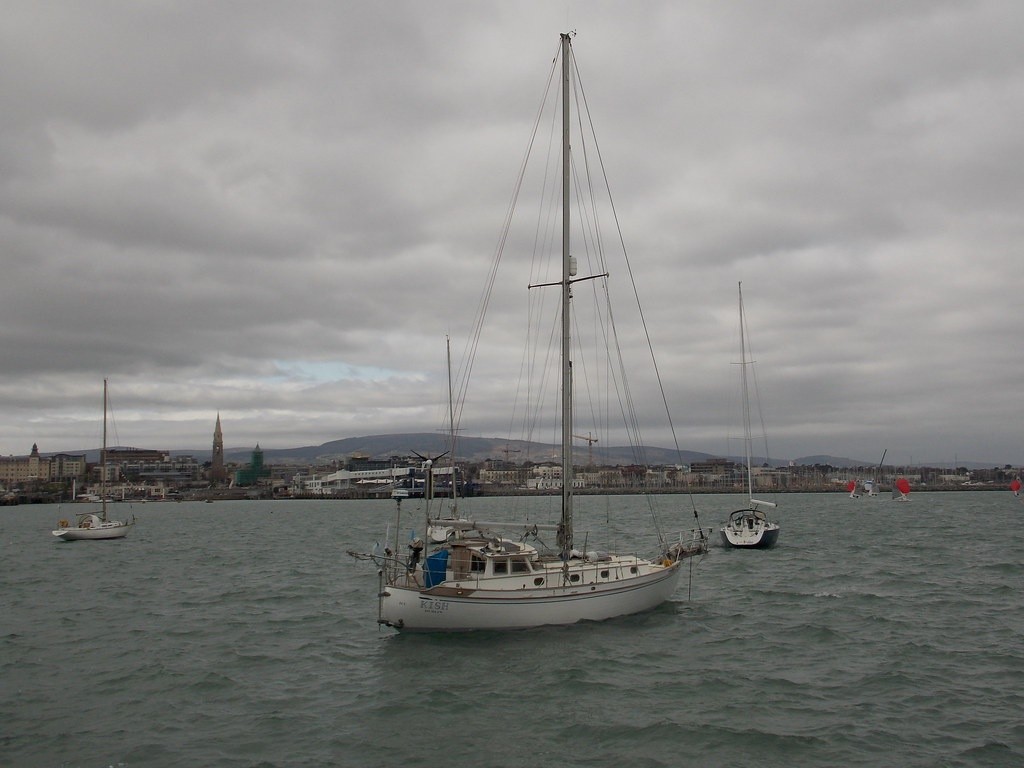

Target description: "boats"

left=722, top=280, right=792, bottom=549
left=845, top=480, right=1021, bottom=503
left=376, top=34, right=714, bottom=632
left=53, top=510, right=135, bottom=542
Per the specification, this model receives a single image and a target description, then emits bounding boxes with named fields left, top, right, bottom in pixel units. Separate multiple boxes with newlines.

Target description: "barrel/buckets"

left=426, top=550, right=448, bottom=588
